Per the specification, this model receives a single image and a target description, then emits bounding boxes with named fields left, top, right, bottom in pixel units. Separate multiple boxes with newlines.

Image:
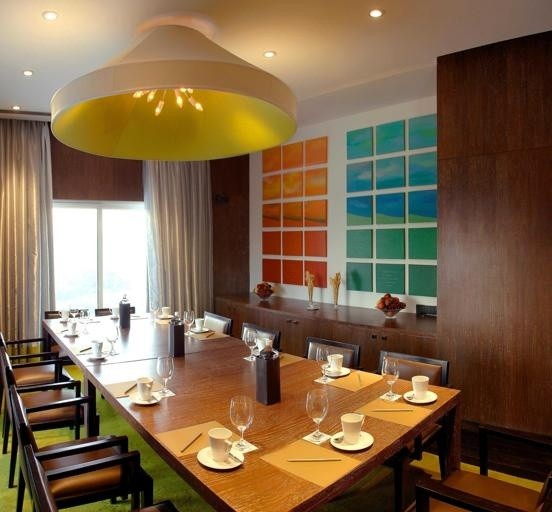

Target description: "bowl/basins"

left=253, top=292, right=277, bottom=304
left=376, top=307, right=407, bottom=320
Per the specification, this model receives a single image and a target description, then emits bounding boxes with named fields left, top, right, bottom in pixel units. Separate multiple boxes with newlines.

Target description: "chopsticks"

left=287, top=457, right=342, bottom=463
left=124, top=383, right=138, bottom=395
left=370, top=408, right=413, bottom=413
left=180, top=431, right=202, bottom=454
left=357, top=373, right=361, bottom=385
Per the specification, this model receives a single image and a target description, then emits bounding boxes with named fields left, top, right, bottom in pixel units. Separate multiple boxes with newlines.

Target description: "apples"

left=375, top=293, right=405, bottom=309
left=255, top=283, right=273, bottom=294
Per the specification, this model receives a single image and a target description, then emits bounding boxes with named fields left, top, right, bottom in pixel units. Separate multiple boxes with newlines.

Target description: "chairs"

left=0, top=328, right=176, bottom=511
left=413, top=422, right=551, bottom=512
left=239, top=321, right=281, bottom=351
left=304, top=336, right=360, bottom=370
left=377, top=349, right=449, bottom=388
left=203, top=310, right=232, bottom=335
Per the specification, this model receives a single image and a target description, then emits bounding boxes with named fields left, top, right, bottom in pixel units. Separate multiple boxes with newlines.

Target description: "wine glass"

left=156, top=357, right=176, bottom=394
left=306, top=389, right=328, bottom=442
left=242, top=326, right=258, bottom=360
left=230, top=396, right=253, bottom=449
left=315, top=346, right=332, bottom=382
left=382, top=356, right=400, bottom=399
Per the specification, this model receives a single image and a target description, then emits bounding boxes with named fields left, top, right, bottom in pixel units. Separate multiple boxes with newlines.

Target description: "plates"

left=253, top=349, right=279, bottom=360
left=195, top=446, right=245, bottom=471
left=321, top=367, right=350, bottom=378
left=403, top=391, right=438, bottom=404
left=329, top=431, right=374, bottom=451
left=131, top=391, right=162, bottom=406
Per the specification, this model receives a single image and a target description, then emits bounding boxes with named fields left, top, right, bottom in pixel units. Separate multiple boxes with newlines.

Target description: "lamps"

left=46, top=22, right=299, bottom=164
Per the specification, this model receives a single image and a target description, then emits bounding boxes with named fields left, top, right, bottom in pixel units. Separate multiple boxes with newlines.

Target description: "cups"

left=136, top=376, right=154, bottom=400
left=257, top=334, right=274, bottom=353
left=59, top=301, right=211, bottom=359
left=208, top=428, right=233, bottom=464
left=411, top=375, right=429, bottom=399
left=339, top=413, right=364, bottom=443
left=327, top=354, right=344, bottom=373
